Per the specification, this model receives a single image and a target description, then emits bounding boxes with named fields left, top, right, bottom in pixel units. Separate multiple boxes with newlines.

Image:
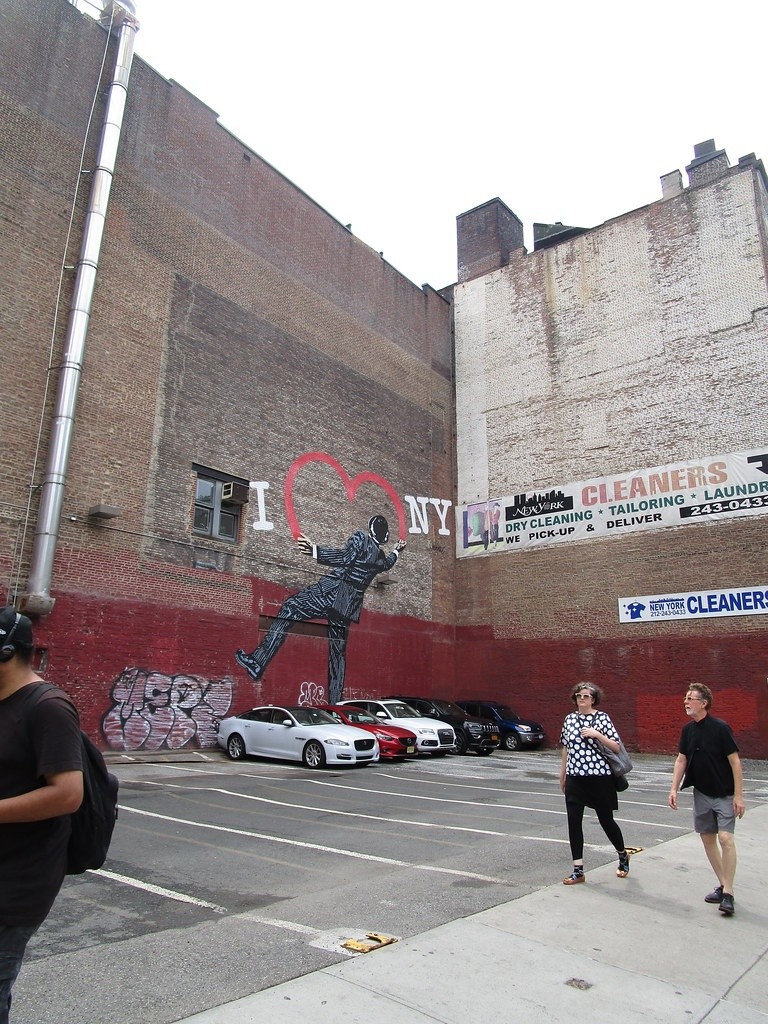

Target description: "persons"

left=559, top=680, right=630, bottom=885
left=668, top=682, right=745, bottom=914
left=0, top=607, right=84, bottom=1024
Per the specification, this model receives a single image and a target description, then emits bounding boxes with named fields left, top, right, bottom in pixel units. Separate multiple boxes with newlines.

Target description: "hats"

left=0, top=608, right=32, bottom=643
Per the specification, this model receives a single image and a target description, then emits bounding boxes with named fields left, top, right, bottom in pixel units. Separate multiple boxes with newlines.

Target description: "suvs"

left=454, top=700, right=546, bottom=751
left=331, top=697, right=457, bottom=759
left=380, top=695, right=501, bottom=756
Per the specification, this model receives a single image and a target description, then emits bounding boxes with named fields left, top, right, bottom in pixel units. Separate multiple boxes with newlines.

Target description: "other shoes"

left=564, top=867, right=585, bottom=884
left=616, top=853, right=630, bottom=877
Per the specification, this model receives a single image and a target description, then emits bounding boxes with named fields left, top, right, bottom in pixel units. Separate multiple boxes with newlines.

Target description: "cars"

left=309, top=705, right=417, bottom=763
left=215, top=703, right=380, bottom=769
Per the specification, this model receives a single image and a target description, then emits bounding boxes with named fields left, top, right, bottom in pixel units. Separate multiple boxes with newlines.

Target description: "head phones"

left=0, top=613, right=22, bottom=663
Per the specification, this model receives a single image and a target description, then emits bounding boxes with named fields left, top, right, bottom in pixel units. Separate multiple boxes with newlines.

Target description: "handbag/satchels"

left=590, top=714, right=633, bottom=777
left=612, top=774, right=629, bottom=792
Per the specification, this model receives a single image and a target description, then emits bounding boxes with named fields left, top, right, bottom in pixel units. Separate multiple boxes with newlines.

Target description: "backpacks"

left=25, top=684, right=119, bottom=874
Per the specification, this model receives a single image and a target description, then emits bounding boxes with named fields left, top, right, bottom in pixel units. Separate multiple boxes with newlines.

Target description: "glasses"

left=575, top=692, right=593, bottom=699
left=683, top=697, right=701, bottom=702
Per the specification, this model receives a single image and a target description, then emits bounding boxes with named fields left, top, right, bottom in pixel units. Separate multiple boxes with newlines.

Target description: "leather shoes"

left=704, top=885, right=724, bottom=903
left=719, top=893, right=734, bottom=913
